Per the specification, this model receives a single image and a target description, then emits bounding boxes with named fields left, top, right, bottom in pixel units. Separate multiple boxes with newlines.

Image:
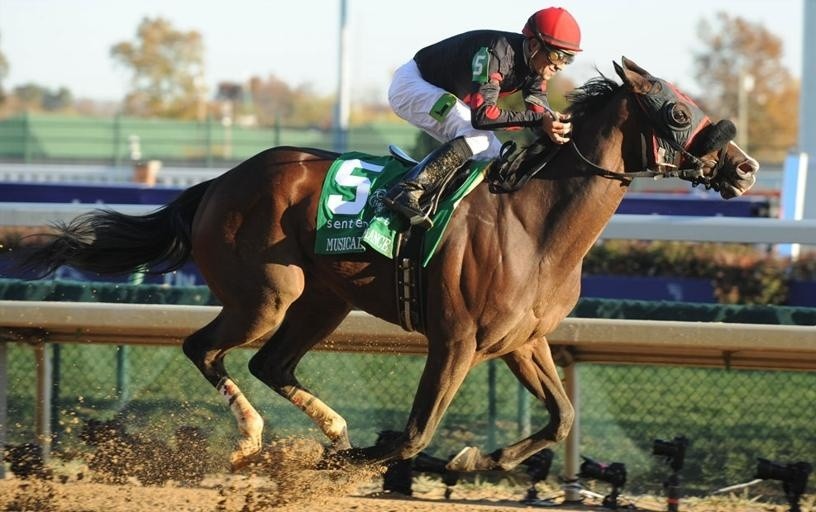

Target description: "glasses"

left=528, top=18, right=574, bottom=66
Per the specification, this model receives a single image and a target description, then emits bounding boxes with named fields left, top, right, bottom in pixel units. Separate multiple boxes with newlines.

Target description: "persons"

left=380, top=6, right=583, bottom=227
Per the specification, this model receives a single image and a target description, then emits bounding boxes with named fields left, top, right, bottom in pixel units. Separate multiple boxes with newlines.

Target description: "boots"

left=384, top=146, right=462, bottom=227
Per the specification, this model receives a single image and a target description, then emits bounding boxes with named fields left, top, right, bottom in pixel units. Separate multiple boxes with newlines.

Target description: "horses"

left=0, top=55, right=760, bottom=482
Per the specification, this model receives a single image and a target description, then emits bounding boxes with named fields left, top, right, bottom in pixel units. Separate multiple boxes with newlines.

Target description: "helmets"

left=521, top=7, right=583, bottom=54
